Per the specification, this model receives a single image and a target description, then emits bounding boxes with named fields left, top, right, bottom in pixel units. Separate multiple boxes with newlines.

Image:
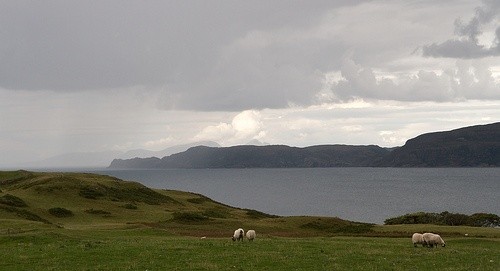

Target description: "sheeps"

left=245, top=229, right=256, bottom=242
left=421, top=233, right=445, bottom=249
left=411, top=233, right=431, bottom=248
left=232, top=227, right=244, bottom=242
